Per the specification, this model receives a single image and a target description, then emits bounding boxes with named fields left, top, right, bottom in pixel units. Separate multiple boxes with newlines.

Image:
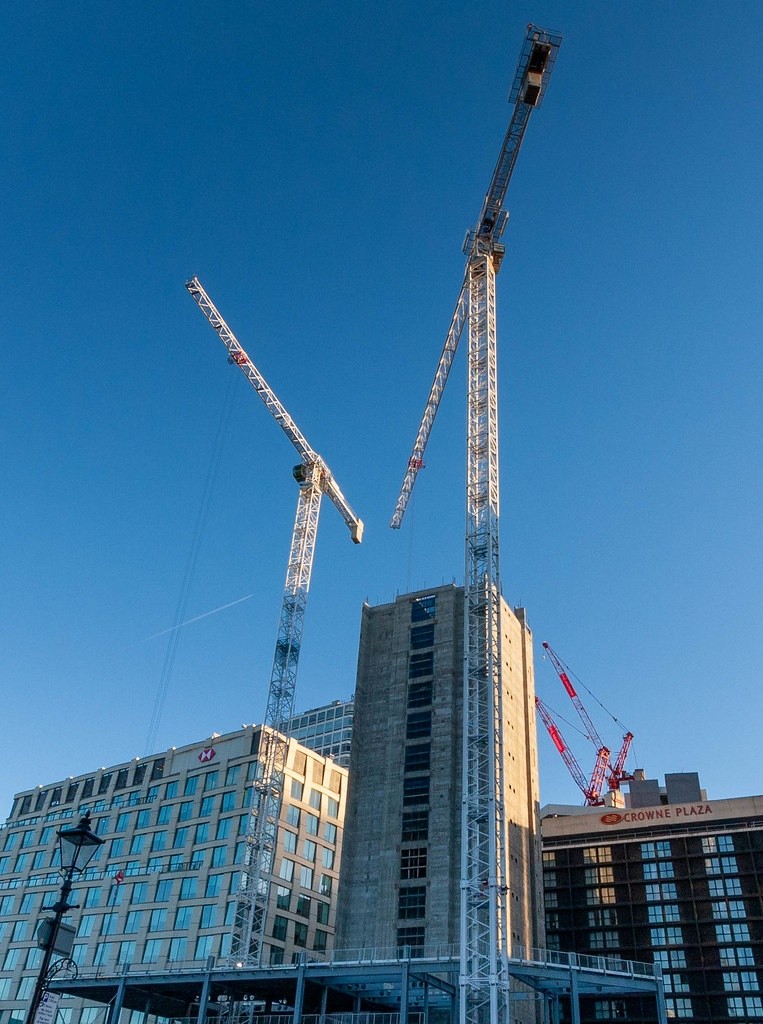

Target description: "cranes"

left=389, top=21, right=563, bottom=1024
left=531, top=636, right=643, bottom=808
left=187, top=277, right=366, bottom=962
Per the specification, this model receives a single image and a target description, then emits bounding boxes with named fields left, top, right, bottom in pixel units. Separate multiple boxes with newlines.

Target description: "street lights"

left=22, top=809, right=104, bottom=1023
